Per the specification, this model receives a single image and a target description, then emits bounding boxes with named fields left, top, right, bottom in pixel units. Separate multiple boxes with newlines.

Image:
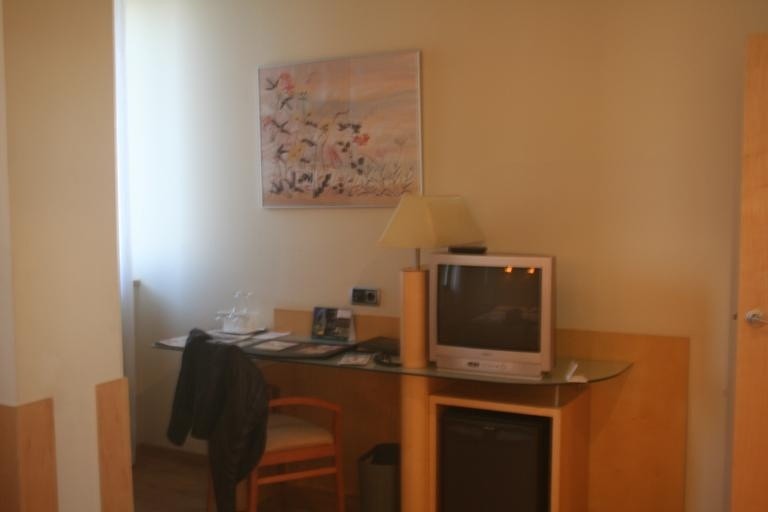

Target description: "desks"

left=149, top=319, right=635, bottom=511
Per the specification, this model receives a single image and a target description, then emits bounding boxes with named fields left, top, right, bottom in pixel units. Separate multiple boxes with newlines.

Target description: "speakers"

left=441, top=409, right=549, bottom=512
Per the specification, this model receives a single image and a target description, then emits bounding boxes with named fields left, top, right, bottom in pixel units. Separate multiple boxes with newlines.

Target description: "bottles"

left=224, top=289, right=260, bottom=333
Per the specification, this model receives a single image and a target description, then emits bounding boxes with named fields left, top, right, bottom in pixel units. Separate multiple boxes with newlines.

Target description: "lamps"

left=376, top=192, right=486, bottom=368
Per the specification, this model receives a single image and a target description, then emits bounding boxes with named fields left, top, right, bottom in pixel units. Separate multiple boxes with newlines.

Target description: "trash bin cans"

left=358, top=442, right=402, bottom=499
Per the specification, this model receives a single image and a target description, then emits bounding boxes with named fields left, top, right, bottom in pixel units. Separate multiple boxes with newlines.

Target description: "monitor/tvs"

left=429, top=252, right=555, bottom=381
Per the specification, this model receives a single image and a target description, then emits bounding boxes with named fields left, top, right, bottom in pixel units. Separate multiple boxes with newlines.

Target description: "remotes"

left=448, top=246, right=487, bottom=254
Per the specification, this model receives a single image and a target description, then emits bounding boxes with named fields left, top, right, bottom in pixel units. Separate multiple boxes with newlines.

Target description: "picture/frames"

left=254, top=50, right=425, bottom=209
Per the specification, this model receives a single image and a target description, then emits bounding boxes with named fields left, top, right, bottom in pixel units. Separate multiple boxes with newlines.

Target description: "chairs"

left=186, top=329, right=347, bottom=512
